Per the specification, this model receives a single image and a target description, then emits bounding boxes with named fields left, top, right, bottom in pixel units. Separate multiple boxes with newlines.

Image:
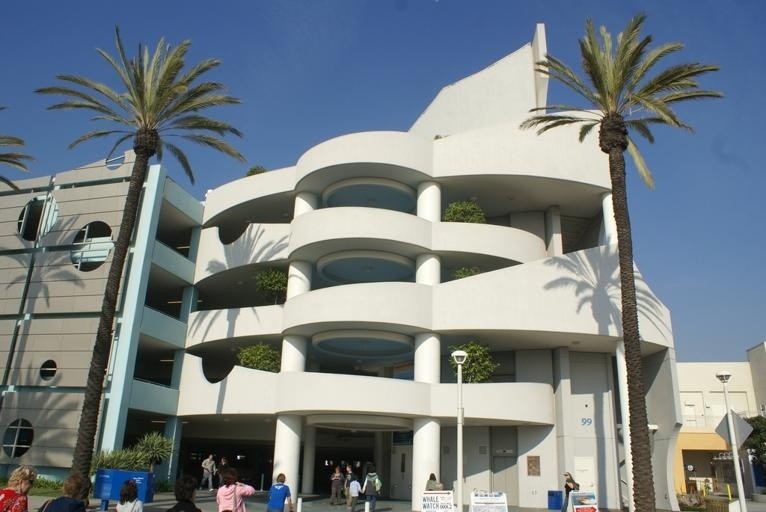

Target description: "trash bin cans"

left=548, top=491, right=562, bottom=509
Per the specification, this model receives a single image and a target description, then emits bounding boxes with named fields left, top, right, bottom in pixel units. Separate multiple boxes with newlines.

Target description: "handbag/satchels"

left=374, top=474, right=382, bottom=491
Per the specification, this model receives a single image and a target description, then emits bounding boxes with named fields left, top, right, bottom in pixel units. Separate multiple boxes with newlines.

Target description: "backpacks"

left=572, top=480, right=580, bottom=490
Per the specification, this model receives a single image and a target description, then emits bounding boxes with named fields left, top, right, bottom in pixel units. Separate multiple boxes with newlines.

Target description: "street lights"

left=449, top=349, right=470, bottom=512
left=714, top=368, right=748, bottom=512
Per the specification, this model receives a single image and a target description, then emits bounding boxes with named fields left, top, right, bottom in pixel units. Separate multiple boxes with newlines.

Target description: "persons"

left=329, top=461, right=381, bottom=512
left=38, top=475, right=91, bottom=512
left=0, top=465, right=37, bottom=512
left=116, top=480, right=144, bottom=512
left=267, top=474, right=293, bottom=512
left=561, top=472, right=577, bottom=512
left=166, top=479, right=202, bottom=512
left=199, top=454, right=216, bottom=492
left=425, top=474, right=438, bottom=492
left=216, top=470, right=255, bottom=512
left=217, top=457, right=229, bottom=488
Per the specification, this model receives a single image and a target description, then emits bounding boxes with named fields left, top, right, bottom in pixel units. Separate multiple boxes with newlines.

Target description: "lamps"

left=712, top=451, right=734, bottom=460
